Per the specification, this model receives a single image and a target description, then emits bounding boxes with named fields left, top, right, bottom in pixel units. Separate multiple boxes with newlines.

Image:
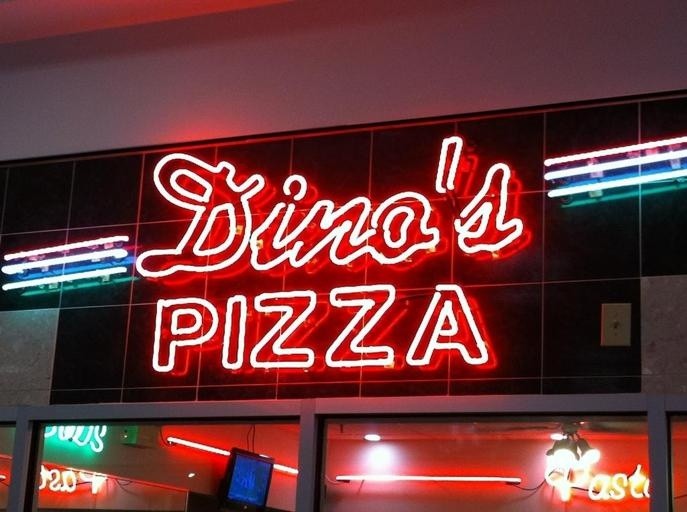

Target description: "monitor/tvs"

left=215, top=448, right=273, bottom=512
